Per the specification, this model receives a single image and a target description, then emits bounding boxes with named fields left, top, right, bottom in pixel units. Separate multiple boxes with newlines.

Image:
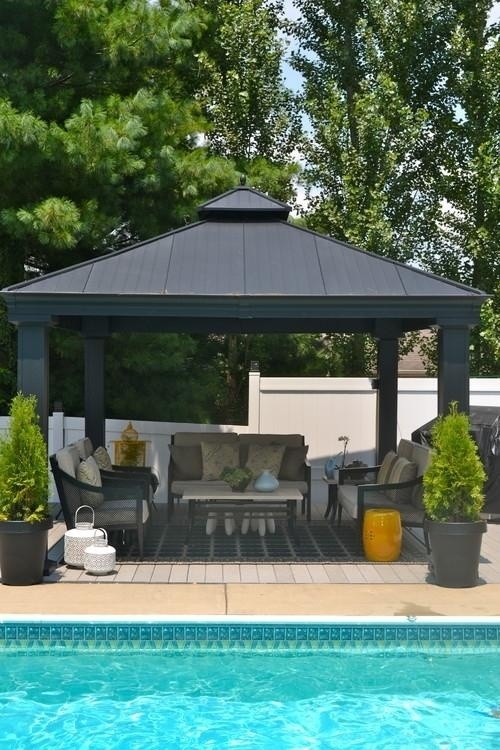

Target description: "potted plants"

left=0, top=389, right=56, bottom=587
left=222, top=465, right=251, bottom=492
left=420, top=399, right=487, bottom=590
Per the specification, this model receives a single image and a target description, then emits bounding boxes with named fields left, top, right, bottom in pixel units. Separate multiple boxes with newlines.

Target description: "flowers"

left=333, top=435, right=350, bottom=471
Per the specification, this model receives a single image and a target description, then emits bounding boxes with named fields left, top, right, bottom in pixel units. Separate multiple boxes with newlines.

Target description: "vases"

left=347, top=460, right=368, bottom=480
left=253, top=467, right=280, bottom=493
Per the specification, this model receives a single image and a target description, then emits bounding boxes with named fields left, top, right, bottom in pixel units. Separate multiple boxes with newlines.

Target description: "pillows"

left=376, top=449, right=417, bottom=505
left=168, top=439, right=309, bottom=482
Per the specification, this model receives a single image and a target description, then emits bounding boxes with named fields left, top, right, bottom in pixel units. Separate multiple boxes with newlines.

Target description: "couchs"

left=336, top=438, right=433, bottom=554
left=166, top=432, right=313, bottom=522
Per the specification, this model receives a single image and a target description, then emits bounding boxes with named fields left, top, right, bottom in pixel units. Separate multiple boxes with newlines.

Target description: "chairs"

left=49, top=437, right=159, bottom=561
